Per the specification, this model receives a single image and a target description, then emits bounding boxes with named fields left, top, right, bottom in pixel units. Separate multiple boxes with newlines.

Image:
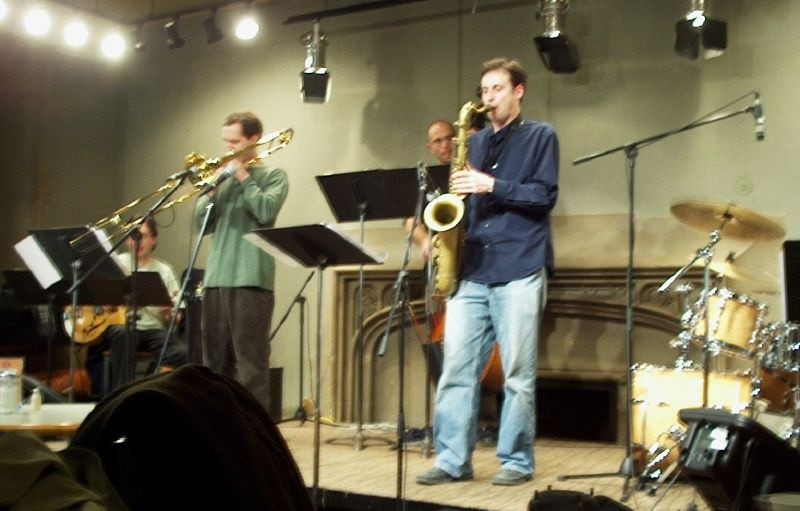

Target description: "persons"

left=405, top=58, right=560, bottom=486
left=193, top=110, right=288, bottom=413
left=104, top=215, right=188, bottom=388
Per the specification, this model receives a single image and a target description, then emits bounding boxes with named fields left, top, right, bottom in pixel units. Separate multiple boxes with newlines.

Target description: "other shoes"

left=416, top=466, right=474, bottom=484
left=491, top=469, right=532, bottom=485
left=481, top=437, right=494, bottom=446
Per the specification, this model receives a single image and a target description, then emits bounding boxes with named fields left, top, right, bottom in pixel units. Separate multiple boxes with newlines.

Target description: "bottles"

left=31, top=387, right=42, bottom=411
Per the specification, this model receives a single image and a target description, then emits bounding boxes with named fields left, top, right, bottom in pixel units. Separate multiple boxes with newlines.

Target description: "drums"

left=690, top=284, right=768, bottom=360
left=631, top=358, right=761, bottom=480
left=758, top=322, right=800, bottom=370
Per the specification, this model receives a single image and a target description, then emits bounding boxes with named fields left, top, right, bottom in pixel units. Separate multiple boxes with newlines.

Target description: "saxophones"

left=423, top=99, right=495, bottom=299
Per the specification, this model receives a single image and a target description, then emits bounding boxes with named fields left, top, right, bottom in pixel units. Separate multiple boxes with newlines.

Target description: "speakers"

left=678, top=407, right=799, bottom=511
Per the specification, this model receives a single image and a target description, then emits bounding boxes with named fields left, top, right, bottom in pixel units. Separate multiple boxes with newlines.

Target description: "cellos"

left=428, top=75, right=505, bottom=424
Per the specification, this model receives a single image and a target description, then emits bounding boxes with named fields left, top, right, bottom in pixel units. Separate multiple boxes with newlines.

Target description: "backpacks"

left=67, top=363, right=313, bottom=511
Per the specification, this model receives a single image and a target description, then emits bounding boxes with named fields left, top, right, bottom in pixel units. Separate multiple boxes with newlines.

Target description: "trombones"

left=70, top=126, right=293, bottom=258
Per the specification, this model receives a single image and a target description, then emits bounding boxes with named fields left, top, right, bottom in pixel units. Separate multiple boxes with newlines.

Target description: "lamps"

left=533, top=0, right=579, bottom=73
left=203, top=7, right=223, bottom=43
left=234, top=0, right=258, bottom=39
left=299, top=19, right=331, bottom=104
left=163, top=15, right=185, bottom=49
left=129, top=21, right=146, bottom=53
left=674, top=0, right=727, bottom=60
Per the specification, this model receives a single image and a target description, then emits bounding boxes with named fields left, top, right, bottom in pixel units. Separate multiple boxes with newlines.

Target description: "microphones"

left=752, top=92, right=765, bottom=141
left=418, top=162, right=440, bottom=202
left=199, top=166, right=237, bottom=196
left=167, top=166, right=199, bottom=182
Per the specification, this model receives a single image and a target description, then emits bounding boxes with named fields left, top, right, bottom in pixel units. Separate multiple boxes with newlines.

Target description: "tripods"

left=250, top=164, right=454, bottom=461
left=558, top=106, right=754, bottom=502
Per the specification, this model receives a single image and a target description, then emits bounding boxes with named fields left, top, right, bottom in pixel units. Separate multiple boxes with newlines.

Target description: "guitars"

left=64, top=304, right=132, bottom=345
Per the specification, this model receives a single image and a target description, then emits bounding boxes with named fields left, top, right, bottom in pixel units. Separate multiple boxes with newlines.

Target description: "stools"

left=100, top=349, right=153, bottom=393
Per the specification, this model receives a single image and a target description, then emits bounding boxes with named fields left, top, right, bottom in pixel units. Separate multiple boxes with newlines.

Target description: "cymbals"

left=669, top=200, right=786, bottom=243
